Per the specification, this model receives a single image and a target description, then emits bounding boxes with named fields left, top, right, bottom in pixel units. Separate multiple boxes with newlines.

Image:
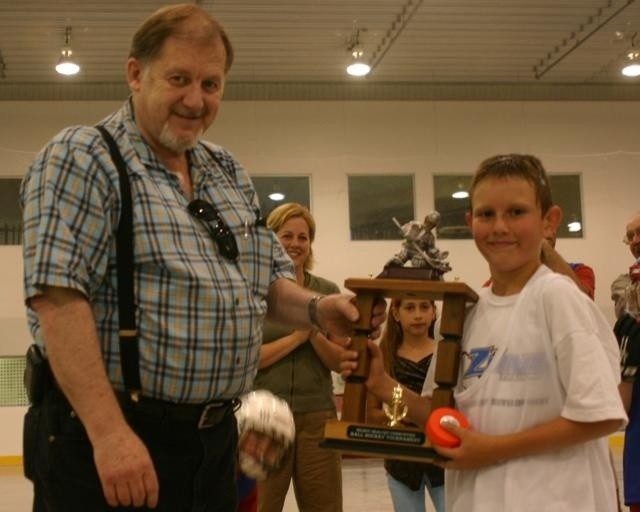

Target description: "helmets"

left=235, top=391, right=295, bottom=480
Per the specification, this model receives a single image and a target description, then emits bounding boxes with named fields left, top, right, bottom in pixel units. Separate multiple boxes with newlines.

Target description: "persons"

left=366, top=295, right=445, bottom=511
left=254, top=203, right=345, bottom=512
left=609, top=214, right=640, bottom=512
left=340, top=154, right=629, bottom=510
left=481, top=224, right=597, bottom=302
left=235, top=387, right=298, bottom=510
left=384, top=208, right=449, bottom=268
left=18, top=5, right=388, bottom=512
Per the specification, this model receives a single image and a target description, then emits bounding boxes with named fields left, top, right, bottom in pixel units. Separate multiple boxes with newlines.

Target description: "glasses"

left=188, top=199, right=239, bottom=260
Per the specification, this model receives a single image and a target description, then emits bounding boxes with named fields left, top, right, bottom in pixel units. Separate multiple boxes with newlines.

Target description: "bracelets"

left=307, top=293, right=327, bottom=328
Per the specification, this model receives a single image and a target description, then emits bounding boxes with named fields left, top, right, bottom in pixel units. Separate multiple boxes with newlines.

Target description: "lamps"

left=609, top=27, right=640, bottom=82
left=341, top=21, right=376, bottom=79
left=55, top=22, right=84, bottom=77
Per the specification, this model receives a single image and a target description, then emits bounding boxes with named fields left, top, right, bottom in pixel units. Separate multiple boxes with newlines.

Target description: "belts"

left=191, top=402, right=230, bottom=430
